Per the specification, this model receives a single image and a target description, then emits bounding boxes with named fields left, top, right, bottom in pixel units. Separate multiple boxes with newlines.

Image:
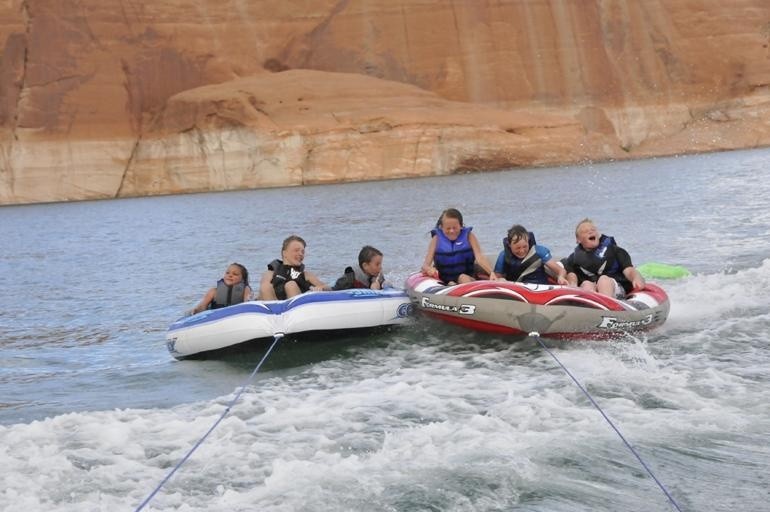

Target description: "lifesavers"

left=638, top=262, right=692, bottom=279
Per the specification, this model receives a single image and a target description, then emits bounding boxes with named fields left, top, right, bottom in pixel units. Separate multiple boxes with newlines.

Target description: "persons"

left=563, top=218, right=646, bottom=302
left=190, top=262, right=255, bottom=314
left=260, top=236, right=332, bottom=303
left=420, top=208, right=498, bottom=287
left=332, top=245, right=393, bottom=291
left=493, top=225, right=568, bottom=285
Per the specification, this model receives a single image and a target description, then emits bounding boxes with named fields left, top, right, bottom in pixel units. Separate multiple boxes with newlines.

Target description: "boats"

left=166, top=284, right=417, bottom=366
left=407, top=264, right=674, bottom=349
